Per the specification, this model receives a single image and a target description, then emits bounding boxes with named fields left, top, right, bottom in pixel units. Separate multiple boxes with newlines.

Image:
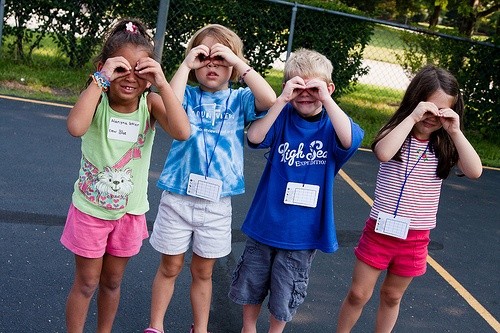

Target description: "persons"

left=227, top=48, right=364, bottom=333
left=60, top=20, right=191, bottom=333
left=144, top=24, right=276, bottom=333
left=337, top=66, right=482, bottom=333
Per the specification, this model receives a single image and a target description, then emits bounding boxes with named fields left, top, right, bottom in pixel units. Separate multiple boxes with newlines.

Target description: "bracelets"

left=241, top=67, right=254, bottom=80
left=89, top=71, right=111, bottom=93
